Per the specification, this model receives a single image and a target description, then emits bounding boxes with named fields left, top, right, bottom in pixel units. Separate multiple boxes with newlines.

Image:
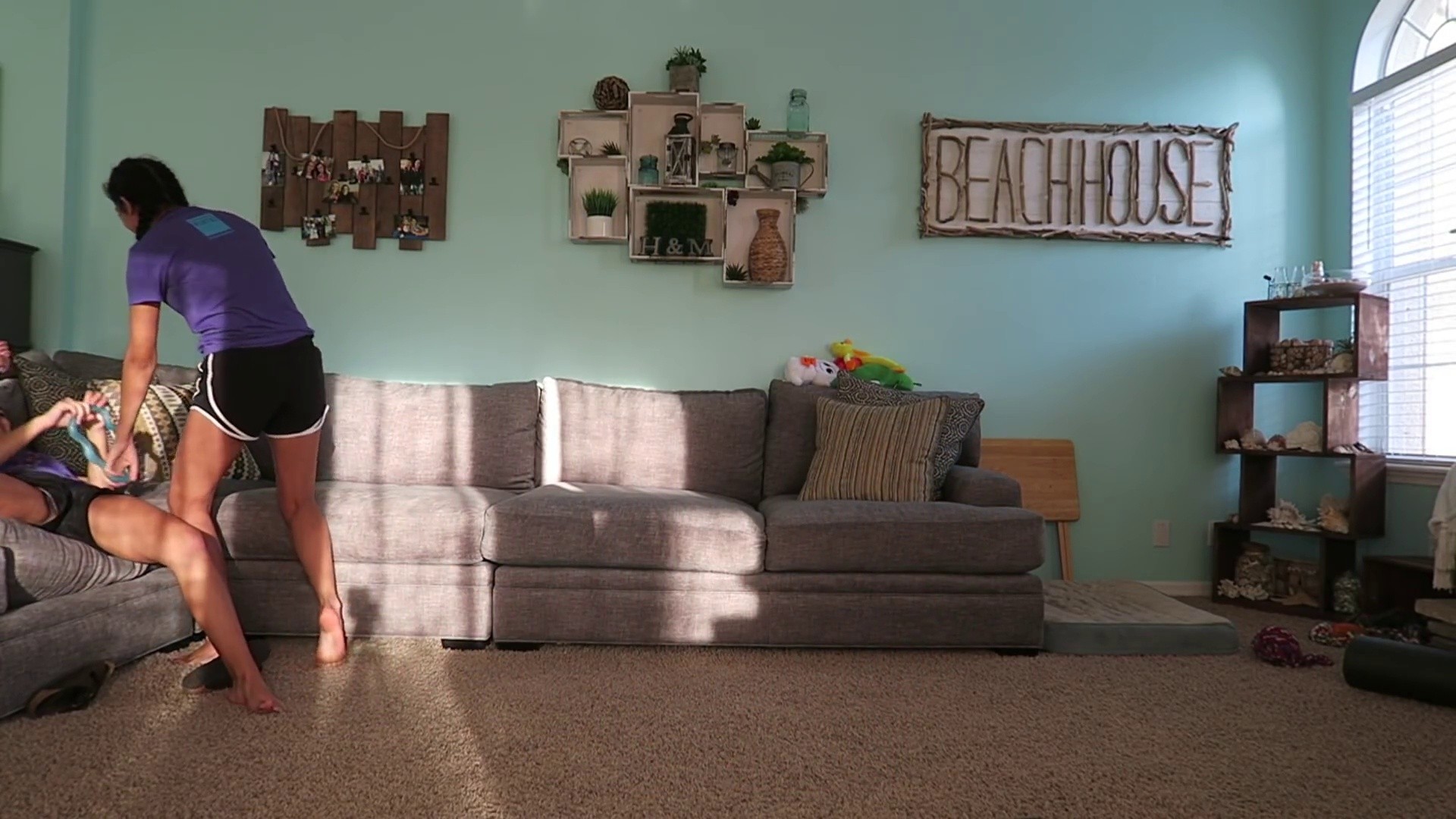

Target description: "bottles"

left=665, top=112, right=695, bottom=154
left=748, top=208, right=787, bottom=282
left=638, top=155, right=659, bottom=186
left=1267, top=259, right=1326, bottom=299
left=786, top=88, right=810, bottom=139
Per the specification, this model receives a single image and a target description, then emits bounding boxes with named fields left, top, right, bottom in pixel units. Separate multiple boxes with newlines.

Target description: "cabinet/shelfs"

left=1212, top=291, right=1390, bottom=623
left=558, top=91, right=830, bottom=287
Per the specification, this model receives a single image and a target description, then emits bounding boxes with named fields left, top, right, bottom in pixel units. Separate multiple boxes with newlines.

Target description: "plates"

left=1304, top=281, right=1367, bottom=296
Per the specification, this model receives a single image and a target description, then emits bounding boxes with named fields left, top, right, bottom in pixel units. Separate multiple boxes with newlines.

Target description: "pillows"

left=12, top=356, right=159, bottom=475
left=795, top=396, right=948, bottom=503
left=90, top=378, right=262, bottom=484
left=830, top=370, right=985, bottom=503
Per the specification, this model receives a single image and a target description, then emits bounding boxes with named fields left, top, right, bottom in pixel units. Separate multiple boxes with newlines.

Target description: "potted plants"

left=581, top=188, right=619, bottom=237
left=666, top=45, right=706, bottom=92
left=749, top=141, right=816, bottom=189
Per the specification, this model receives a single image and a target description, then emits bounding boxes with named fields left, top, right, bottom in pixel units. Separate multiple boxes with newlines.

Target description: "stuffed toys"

left=784, top=338, right=924, bottom=391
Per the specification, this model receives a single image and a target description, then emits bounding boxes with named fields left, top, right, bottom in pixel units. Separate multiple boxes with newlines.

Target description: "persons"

left=0, top=391, right=286, bottom=714
left=100, top=157, right=346, bottom=664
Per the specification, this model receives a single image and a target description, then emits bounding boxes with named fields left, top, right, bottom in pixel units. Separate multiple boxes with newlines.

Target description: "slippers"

left=29, top=660, right=118, bottom=719
left=183, top=655, right=263, bottom=690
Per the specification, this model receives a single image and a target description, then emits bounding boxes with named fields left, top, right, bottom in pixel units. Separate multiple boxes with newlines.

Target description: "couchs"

left=0, top=364, right=1046, bottom=717
